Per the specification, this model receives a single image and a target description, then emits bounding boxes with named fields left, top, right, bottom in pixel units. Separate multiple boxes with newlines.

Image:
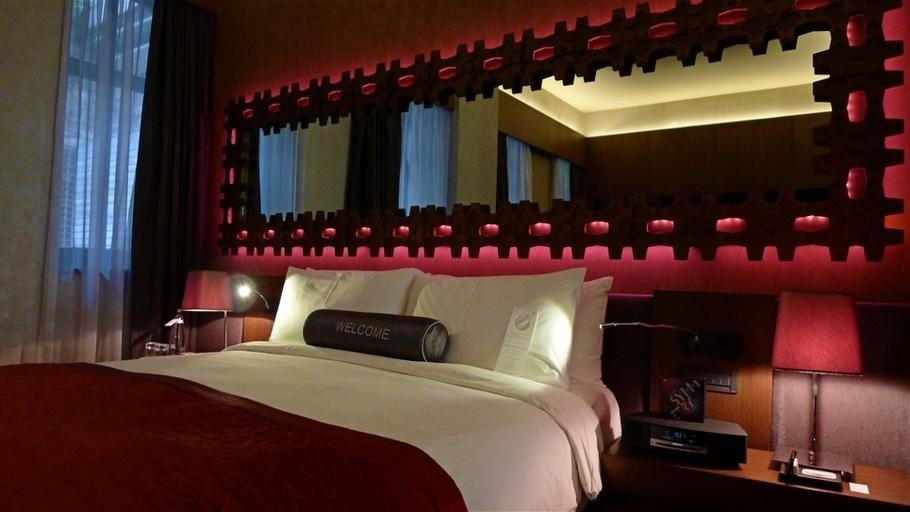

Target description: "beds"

left=0, top=270, right=621, bottom=511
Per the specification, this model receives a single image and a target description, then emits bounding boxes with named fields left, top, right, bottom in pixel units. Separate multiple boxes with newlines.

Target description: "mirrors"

left=248, top=26, right=833, bottom=212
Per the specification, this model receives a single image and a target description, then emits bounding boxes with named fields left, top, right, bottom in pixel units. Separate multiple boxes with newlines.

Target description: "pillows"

left=569, top=274, right=615, bottom=384
left=409, top=265, right=589, bottom=391
left=269, top=265, right=427, bottom=360
left=302, top=307, right=452, bottom=364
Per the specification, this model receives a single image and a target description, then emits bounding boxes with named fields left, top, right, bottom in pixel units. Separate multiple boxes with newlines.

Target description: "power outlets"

left=696, top=368, right=740, bottom=395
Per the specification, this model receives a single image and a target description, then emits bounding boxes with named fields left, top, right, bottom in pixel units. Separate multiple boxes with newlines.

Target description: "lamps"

left=772, top=289, right=865, bottom=482
left=182, top=269, right=234, bottom=354
